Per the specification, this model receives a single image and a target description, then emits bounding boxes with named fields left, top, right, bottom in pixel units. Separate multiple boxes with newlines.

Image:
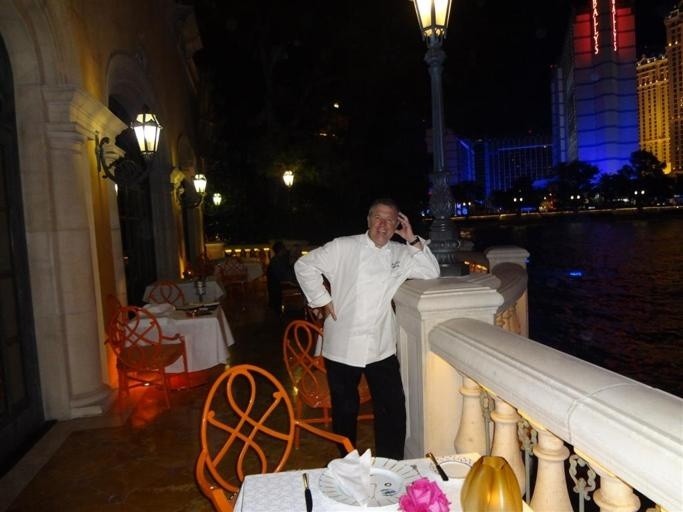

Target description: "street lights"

left=462, top=200, right=472, bottom=217
left=512, top=194, right=523, bottom=216
left=410, top=0, right=478, bottom=278
left=633, top=190, right=645, bottom=209
left=570, top=194, right=581, bottom=212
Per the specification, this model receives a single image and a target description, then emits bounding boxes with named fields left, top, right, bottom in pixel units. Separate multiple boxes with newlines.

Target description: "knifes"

left=427, top=450, right=447, bottom=480
left=301, top=472, right=314, bottom=512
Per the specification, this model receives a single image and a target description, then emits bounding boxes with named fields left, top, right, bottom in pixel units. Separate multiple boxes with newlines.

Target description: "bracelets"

left=408, top=236, right=421, bottom=247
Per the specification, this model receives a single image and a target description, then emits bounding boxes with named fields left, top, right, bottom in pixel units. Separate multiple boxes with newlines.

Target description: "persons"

left=291, top=195, right=442, bottom=462
left=288, top=243, right=303, bottom=258
left=267, top=240, right=294, bottom=312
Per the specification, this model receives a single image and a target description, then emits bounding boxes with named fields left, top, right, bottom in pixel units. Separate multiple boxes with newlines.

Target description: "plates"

left=431, top=453, right=476, bottom=481
left=319, top=456, right=417, bottom=509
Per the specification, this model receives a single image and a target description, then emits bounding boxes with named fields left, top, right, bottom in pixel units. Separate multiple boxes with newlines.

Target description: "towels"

left=322, top=444, right=382, bottom=508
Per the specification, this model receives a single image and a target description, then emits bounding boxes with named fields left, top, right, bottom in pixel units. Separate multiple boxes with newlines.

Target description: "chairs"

left=282, top=320, right=375, bottom=458
left=107, top=251, right=328, bottom=409
left=196, top=365, right=357, bottom=512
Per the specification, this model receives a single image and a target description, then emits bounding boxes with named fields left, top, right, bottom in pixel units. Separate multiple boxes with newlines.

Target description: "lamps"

left=459, top=453, right=523, bottom=512
left=92, top=112, right=165, bottom=191
left=174, top=172, right=210, bottom=214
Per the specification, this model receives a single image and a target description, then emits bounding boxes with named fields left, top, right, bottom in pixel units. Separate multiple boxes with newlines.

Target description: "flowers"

left=398, top=473, right=451, bottom=512
